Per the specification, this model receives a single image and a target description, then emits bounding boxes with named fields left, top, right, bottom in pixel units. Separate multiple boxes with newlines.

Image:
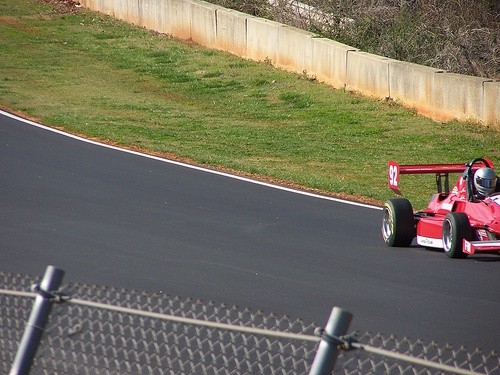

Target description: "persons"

left=468, top=167, right=497, bottom=203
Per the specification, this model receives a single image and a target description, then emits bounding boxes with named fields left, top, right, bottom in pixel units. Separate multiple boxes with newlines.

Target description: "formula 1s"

left=381, top=157, right=500, bottom=259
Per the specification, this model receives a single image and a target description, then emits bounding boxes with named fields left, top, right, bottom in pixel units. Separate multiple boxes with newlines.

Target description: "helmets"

left=474, top=168, right=497, bottom=196
